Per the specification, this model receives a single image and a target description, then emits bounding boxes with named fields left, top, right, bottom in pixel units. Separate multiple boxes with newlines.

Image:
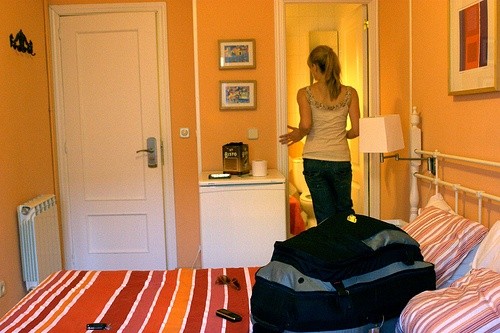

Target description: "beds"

left=0, top=105, right=500, bottom=333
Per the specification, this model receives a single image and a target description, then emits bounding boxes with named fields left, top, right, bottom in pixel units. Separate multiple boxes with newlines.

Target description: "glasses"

left=215, top=274, right=241, bottom=289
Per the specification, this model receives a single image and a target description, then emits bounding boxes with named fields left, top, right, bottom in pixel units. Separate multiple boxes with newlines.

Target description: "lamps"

left=358, top=112, right=439, bottom=176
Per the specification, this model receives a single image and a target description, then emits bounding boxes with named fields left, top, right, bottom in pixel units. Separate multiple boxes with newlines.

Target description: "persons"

left=279, top=44, right=361, bottom=225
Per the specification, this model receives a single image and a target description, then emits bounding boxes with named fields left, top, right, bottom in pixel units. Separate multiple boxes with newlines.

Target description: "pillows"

left=397, top=192, right=500, bottom=333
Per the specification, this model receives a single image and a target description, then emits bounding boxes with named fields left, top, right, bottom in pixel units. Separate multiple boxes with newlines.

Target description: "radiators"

left=13, top=194, right=64, bottom=293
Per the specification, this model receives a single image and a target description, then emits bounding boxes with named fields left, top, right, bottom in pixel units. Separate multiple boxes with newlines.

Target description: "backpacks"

left=251, top=214, right=436, bottom=333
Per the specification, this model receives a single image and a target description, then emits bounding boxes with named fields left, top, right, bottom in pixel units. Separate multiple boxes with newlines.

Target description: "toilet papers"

left=251, top=160, right=268, bottom=176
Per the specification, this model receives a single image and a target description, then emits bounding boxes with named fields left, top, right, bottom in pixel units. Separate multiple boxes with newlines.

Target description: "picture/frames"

left=218, top=38, right=256, bottom=70
left=219, top=79, right=258, bottom=111
left=447, top=0, right=500, bottom=97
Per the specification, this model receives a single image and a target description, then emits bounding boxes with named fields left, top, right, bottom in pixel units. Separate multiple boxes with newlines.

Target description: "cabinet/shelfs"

left=198, top=167, right=288, bottom=270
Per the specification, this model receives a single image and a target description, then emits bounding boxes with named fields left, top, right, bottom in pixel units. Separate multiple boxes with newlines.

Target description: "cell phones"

left=216, top=309, right=241, bottom=322
left=86, top=323, right=105, bottom=330
